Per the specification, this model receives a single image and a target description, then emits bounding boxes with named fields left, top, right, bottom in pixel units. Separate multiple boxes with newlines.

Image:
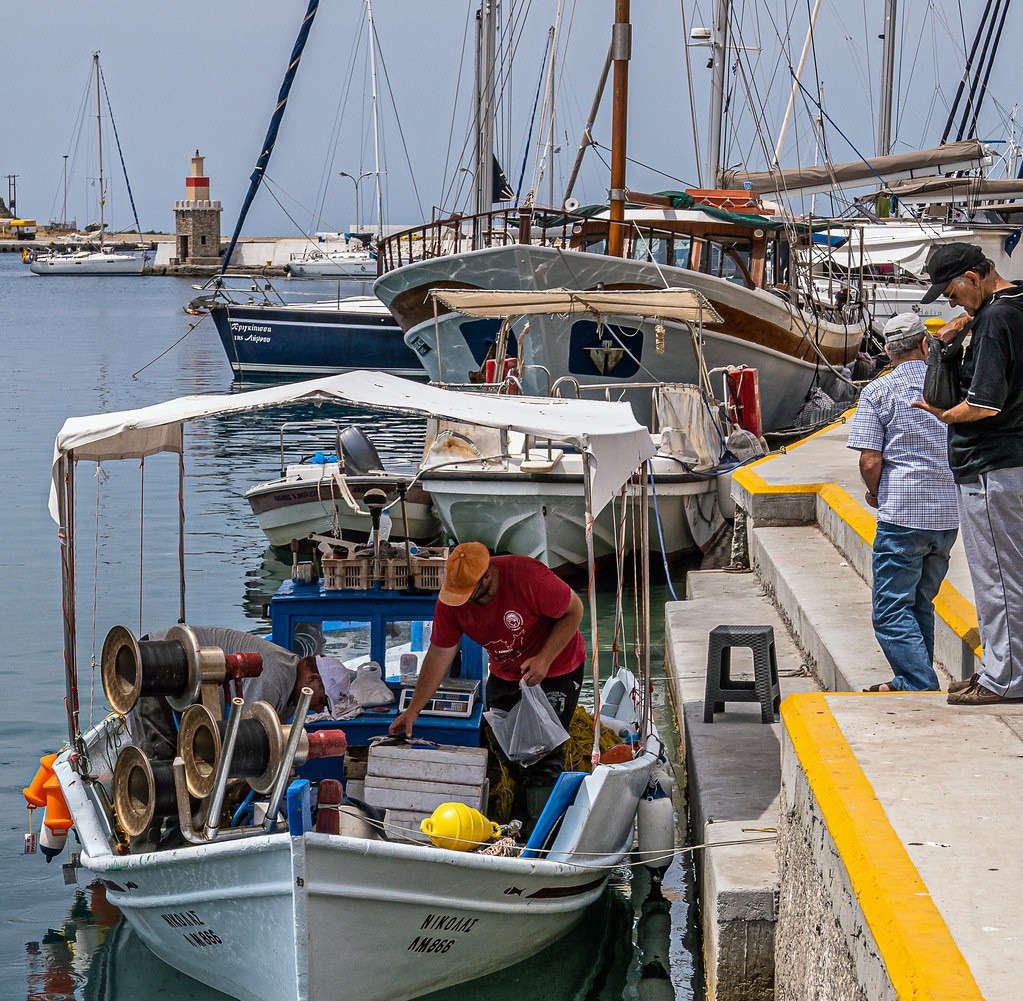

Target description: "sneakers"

left=948, top=671, right=981, bottom=693
left=946, top=683, right=1023, bottom=705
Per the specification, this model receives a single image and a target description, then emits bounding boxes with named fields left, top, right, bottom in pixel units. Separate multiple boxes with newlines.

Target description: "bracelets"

left=866, top=489, right=876, bottom=499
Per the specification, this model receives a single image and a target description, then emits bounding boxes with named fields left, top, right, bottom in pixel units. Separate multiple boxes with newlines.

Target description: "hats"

left=920, top=242, right=986, bottom=304
left=315, top=654, right=350, bottom=718
left=881, top=311, right=927, bottom=343
left=438, top=541, right=488, bottom=606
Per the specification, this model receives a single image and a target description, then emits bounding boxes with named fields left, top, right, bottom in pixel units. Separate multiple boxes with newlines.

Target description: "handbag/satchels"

left=349, top=661, right=395, bottom=707
left=483, top=679, right=571, bottom=768
left=923, top=337, right=964, bottom=409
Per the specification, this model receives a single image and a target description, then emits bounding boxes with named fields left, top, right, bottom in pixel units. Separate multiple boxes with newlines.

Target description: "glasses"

left=469, top=584, right=483, bottom=601
left=944, top=277, right=962, bottom=301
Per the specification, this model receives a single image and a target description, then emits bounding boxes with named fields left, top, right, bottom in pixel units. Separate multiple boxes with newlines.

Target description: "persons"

left=911, top=241, right=1023, bottom=706
left=835, top=287, right=853, bottom=314
left=845, top=312, right=961, bottom=693
left=390, top=541, right=589, bottom=832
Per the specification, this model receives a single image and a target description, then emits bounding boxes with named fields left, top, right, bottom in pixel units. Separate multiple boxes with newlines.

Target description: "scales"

left=398, top=677, right=481, bottom=718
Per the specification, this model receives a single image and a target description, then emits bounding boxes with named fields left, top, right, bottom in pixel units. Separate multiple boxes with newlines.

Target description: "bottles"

left=599, top=714, right=638, bottom=744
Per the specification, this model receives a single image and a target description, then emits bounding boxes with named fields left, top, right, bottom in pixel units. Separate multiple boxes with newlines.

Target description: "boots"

left=525, top=785, right=552, bottom=844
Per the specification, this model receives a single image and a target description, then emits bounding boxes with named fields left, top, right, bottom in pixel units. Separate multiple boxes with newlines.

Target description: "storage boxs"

left=367, top=741, right=489, bottom=786
left=382, top=809, right=432, bottom=842
left=363, top=775, right=485, bottom=814
left=390, top=547, right=450, bottom=590
left=321, top=548, right=389, bottom=589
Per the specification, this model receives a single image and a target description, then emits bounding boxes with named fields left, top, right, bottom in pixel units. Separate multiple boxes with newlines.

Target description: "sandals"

left=863, top=682, right=898, bottom=691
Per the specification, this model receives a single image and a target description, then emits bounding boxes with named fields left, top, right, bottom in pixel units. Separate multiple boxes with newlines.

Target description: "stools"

left=703, top=624, right=782, bottom=724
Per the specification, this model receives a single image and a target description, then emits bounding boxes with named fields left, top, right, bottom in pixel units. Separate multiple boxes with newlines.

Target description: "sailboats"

left=30, top=0, right=1022, bottom=581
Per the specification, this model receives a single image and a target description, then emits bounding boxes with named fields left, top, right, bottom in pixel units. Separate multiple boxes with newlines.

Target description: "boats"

left=45, top=373, right=659, bottom=1000
